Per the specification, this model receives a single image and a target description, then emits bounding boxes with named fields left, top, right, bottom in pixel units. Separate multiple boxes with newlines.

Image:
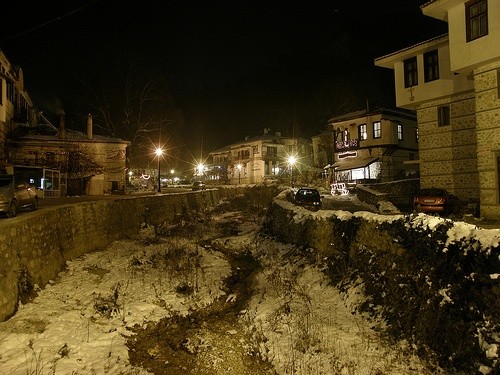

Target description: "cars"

left=191, top=181, right=206, bottom=191
left=413, top=188, right=455, bottom=217
left=0, top=173, right=39, bottom=218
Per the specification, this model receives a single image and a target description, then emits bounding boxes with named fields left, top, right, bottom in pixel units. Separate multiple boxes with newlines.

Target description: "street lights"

left=286, top=155, right=298, bottom=189
left=236, top=163, right=242, bottom=184
left=154, top=147, right=163, bottom=193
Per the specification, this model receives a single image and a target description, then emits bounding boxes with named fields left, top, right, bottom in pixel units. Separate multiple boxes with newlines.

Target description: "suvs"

left=292, top=188, right=324, bottom=212
